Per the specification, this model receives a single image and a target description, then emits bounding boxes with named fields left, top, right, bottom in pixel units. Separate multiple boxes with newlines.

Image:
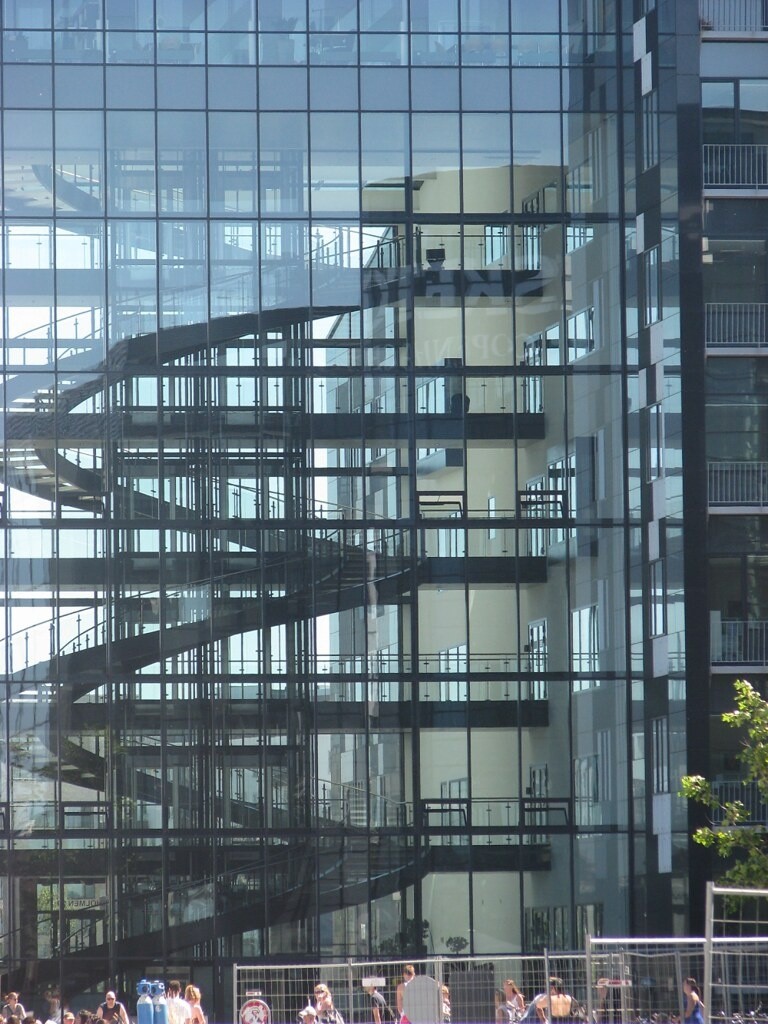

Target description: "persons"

left=671, top=978, right=705, bottom=1024
left=165, top=980, right=204, bottom=1024
left=296, top=984, right=344, bottom=1024
left=364, top=965, right=450, bottom=1024
left=494, top=976, right=589, bottom=1024
left=0, top=989, right=129, bottom=1024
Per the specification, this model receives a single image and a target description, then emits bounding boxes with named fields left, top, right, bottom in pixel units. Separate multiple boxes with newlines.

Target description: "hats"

left=65, top=1012, right=75, bottom=1020
left=298, top=1006, right=316, bottom=1018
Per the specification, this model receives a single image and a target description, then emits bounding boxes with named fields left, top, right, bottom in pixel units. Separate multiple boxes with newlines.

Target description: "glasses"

left=11, top=997, right=18, bottom=1002
left=313, top=990, right=324, bottom=994
left=106, top=998, right=115, bottom=1001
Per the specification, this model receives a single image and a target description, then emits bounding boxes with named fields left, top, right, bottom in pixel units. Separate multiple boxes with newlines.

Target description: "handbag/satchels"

left=382, top=1006, right=395, bottom=1021
